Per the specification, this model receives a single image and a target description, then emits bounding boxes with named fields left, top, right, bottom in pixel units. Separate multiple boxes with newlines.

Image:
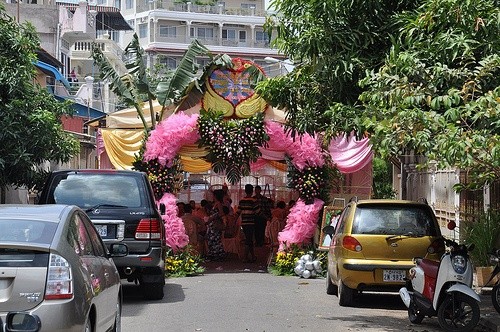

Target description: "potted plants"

left=463, top=211, right=497, bottom=287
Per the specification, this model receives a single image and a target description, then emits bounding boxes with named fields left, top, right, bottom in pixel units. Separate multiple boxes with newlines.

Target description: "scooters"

left=399, top=220, right=483, bottom=332
left=484, top=250, right=500, bottom=316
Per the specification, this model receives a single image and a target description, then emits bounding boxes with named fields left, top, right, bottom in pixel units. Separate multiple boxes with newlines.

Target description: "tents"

left=83, top=58, right=373, bottom=203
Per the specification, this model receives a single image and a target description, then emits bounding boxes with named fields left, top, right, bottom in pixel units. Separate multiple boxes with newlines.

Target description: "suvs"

left=37, top=169, right=167, bottom=295
left=322, top=195, right=445, bottom=306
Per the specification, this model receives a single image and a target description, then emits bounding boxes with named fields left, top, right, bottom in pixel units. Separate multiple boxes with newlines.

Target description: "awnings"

left=31, top=59, right=72, bottom=95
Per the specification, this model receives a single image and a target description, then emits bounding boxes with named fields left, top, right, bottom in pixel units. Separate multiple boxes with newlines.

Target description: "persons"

left=176, top=183, right=295, bottom=262
left=323, top=213, right=341, bottom=246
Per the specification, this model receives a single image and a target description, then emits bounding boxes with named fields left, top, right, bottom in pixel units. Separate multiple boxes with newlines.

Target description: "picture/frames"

left=318, top=205, right=346, bottom=251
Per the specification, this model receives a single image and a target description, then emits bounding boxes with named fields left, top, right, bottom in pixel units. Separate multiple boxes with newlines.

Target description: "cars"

left=0, top=202, right=122, bottom=332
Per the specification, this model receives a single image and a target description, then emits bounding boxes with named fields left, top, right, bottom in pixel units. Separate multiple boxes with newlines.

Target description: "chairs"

left=182, top=217, right=197, bottom=243
left=265, top=218, right=287, bottom=267
